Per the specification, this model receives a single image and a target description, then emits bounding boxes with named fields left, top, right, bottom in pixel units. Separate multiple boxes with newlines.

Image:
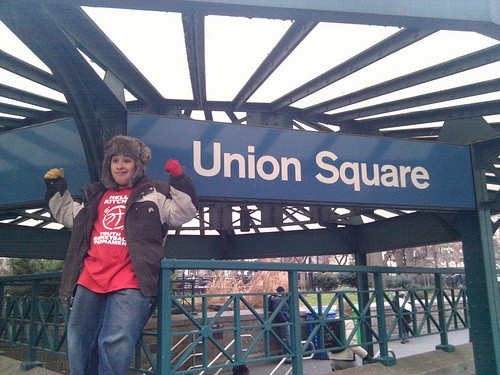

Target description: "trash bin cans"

left=306, top=309, right=338, bottom=360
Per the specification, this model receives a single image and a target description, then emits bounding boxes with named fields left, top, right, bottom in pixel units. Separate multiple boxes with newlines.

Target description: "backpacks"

left=268, top=294, right=281, bottom=311
left=323, top=317, right=350, bottom=353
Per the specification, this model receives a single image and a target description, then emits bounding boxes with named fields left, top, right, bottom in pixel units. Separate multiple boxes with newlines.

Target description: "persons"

left=391, top=291, right=413, bottom=344
left=266, top=287, right=292, bottom=364
left=42, top=135, right=199, bottom=375
left=325, top=300, right=373, bottom=371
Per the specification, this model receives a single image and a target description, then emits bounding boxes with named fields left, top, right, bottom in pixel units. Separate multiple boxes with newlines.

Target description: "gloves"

left=363, top=354, right=373, bottom=362
left=165, top=159, right=183, bottom=176
left=43, top=168, right=66, bottom=201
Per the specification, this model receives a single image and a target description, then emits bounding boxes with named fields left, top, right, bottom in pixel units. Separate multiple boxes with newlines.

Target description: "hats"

left=336, top=301, right=352, bottom=311
left=399, top=291, right=405, bottom=296
left=101, top=135, right=151, bottom=191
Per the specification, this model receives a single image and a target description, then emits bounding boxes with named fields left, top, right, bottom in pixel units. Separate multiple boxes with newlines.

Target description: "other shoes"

left=286, top=358, right=292, bottom=363
left=402, top=340, right=410, bottom=343
